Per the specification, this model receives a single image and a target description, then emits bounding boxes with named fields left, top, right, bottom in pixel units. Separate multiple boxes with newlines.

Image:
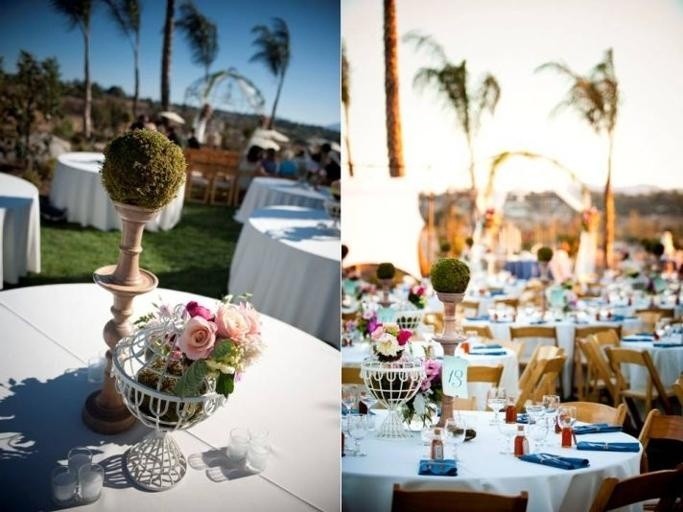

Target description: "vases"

left=105, top=311, right=228, bottom=496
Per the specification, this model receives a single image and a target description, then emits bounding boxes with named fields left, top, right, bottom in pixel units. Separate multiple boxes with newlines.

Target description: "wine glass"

left=444, top=416, right=467, bottom=462
left=653, top=322, right=683, bottom=344
left=341, top=383, right=379, bottom=459
left=487, top=388, right=576, bottom=458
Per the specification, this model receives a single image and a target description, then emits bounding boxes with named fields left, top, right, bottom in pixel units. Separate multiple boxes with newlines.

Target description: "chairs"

left=187, top=148, right=258, bottom=208
left=342, top=257, right=683, bottom=511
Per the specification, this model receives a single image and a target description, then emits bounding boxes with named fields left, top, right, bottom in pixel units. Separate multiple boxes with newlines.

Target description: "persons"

left=117, top=99, right=340, bottom=187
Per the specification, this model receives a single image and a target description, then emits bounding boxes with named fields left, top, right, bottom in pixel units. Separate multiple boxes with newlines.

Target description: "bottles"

left=595, top=296, right=631, bottom=321
left=430, top=428, right=443, bottom=459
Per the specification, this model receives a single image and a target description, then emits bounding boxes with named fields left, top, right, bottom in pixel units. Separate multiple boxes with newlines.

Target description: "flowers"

left=128, top=290, right=273, bottom=400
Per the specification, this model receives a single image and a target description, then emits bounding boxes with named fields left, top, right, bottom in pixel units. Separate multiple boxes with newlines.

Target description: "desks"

left=0, top=282, right=340, bottom=511
left=232, top=176, right=334, bottom=225
left=224, top=204, right=339, bottom=350
left=1, top=167, right=40, bottom=288
left=51, top=150, right=186, bottom=231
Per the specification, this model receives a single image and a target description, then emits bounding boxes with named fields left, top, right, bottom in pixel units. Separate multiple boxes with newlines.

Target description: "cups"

left=50, top=448, right=106, bottom=504
left=225, top=423, right=274, bottom=472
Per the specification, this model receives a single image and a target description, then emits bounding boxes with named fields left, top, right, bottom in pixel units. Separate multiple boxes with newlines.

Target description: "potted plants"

left=651, top=241, right=665, bottom=267
left=537, top=248, right=554, bottom=314
left=83, top=125, right=188, bottom=434
